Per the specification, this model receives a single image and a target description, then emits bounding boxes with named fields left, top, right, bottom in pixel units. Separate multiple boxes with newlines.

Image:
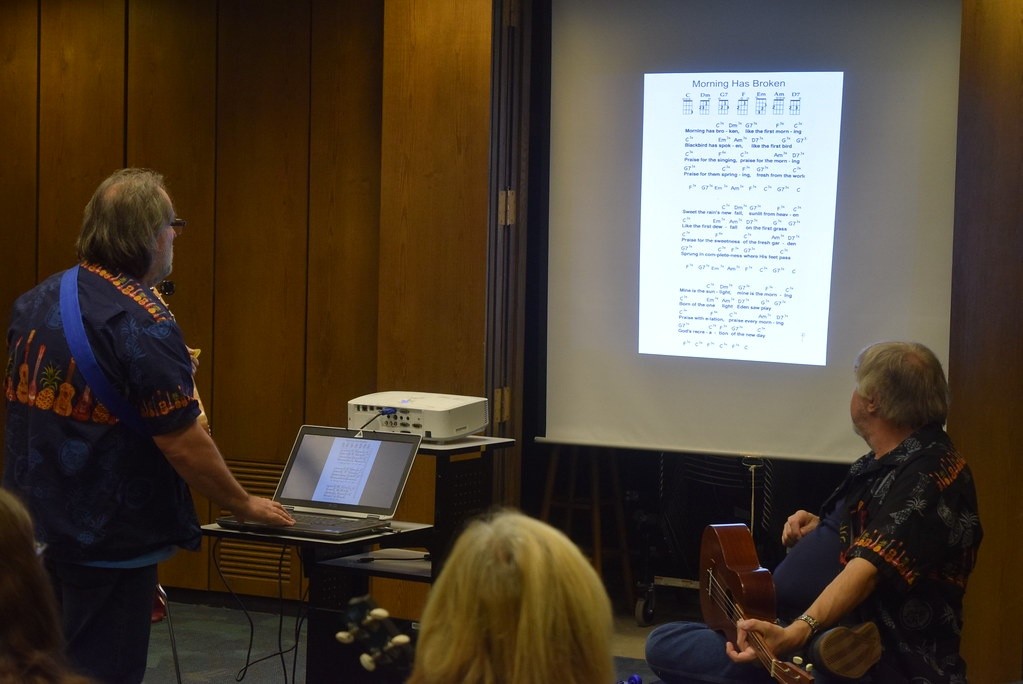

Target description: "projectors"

left=347, top=390, right=489, bottom=445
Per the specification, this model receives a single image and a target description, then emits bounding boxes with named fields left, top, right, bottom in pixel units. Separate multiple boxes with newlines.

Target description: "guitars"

left=698, top=522, right=813, bottom=683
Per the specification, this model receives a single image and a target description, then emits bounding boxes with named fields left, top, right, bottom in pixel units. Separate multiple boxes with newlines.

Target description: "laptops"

left=215, top=424, right=423, bottom=542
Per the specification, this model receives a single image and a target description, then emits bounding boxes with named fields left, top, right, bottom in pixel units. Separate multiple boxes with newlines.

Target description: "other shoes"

left=805, top=621, right=883, bottom=681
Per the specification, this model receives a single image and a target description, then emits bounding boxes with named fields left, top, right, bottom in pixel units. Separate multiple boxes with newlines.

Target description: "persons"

left=0, top=488, right=98, bottom=684
left=645, top=341, right=984, bottom=684
left=407, top=509, right=616, bottom=684
left=3, top=168, right=297, bottom=684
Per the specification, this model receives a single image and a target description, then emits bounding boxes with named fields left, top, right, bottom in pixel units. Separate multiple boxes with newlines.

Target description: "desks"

left=199, top=520, right=431, bottom=683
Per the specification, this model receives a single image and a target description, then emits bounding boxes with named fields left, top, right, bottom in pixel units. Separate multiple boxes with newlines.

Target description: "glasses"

left=169, top=218, right=187, bottom=236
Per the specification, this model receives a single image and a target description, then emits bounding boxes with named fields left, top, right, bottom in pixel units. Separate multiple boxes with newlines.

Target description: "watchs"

left=794, top=614, right=822, bottom=639
